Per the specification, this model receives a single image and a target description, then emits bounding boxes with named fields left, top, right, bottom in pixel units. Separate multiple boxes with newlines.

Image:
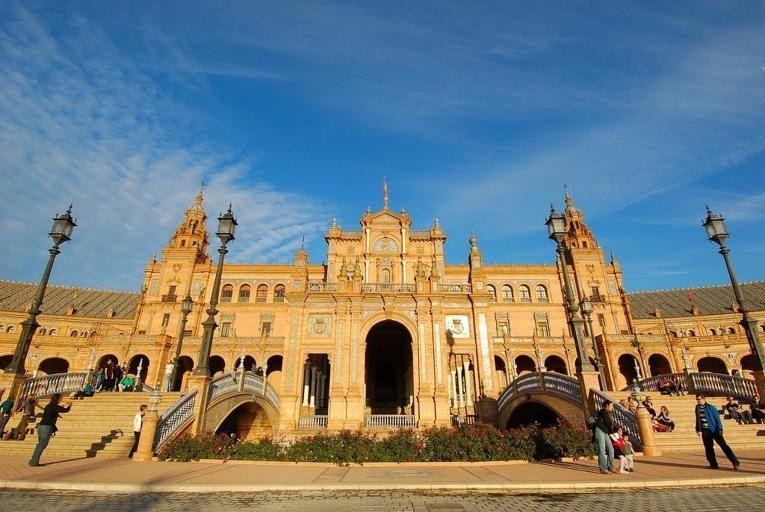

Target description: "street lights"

left=0, top=202, right=80, bottom=374
left=700, top=201, right=764, bottom=374
left=188, top=200, right=240, bottom=380
left=167, top=288, right=194, bottom=391
left=575, top=288, right=609, bottom=394
left=542, top=201, right=604, bottom=376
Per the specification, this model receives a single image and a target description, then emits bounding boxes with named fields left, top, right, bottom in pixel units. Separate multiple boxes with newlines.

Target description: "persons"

left=28, top=394, right=72, bottom=466
left=256, top=367, right=263, bottom=376
left=656, top=377, right=685, bottom=397
left=0, top=394, right=36, bottom=440
left=695, top=393, right=742, bottom=470
left=726, top=395, right=765, bottom=424
left=128, top=404, right=147, bottom=458
left=594, top=400, right=636, bottom=474
left=163, top=360, right=175, bottom=392
left=619, top=396, right=675, bottom=432
left=79, top=359, right=129, bottom=400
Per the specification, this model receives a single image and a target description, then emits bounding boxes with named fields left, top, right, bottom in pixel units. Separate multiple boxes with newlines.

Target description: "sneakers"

left=600, top=467, right=634, bottom=475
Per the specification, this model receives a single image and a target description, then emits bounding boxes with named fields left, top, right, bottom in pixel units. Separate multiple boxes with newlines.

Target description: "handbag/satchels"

left=52, top=426, right=59, bottom=433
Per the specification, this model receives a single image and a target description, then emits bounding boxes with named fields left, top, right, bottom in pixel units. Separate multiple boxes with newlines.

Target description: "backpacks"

left=585, top=414, right=597, bottom=432
